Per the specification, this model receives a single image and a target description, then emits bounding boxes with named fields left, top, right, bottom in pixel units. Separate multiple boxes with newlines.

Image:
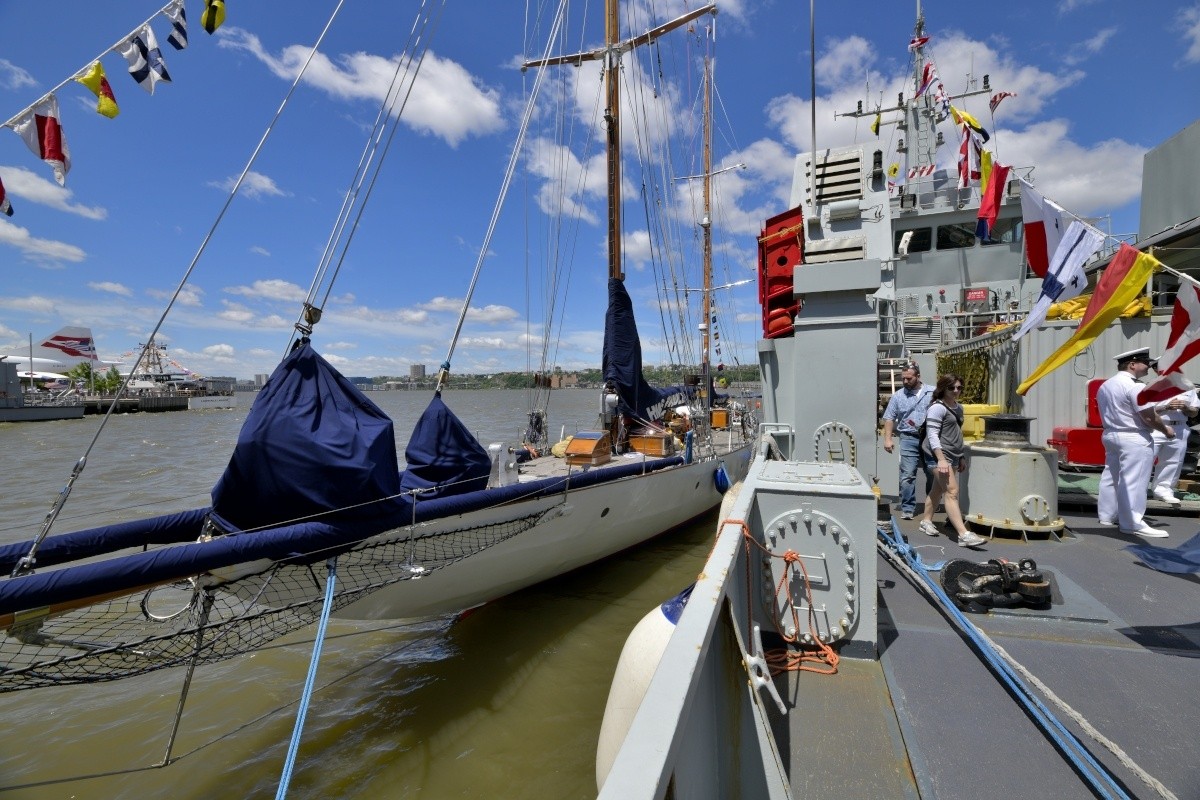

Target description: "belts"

left=903, top=431, right=913, bottom=436
left=1170, top=421, right=1180, bottom=425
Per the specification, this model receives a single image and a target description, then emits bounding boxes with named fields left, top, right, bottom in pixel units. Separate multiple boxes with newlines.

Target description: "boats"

left=0, top=355, right=87, bottom=422
left=115, top=337, right=189, bottom=397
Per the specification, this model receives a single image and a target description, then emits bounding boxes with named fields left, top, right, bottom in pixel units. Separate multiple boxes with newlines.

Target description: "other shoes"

left=935, top=506, right=941, bottom=513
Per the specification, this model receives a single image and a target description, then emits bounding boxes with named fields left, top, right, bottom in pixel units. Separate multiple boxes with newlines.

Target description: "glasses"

left=946, top=385, right=964, bottom=391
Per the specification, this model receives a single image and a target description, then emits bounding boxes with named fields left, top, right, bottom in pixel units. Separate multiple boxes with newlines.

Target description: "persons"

left=146, top=389, right=150, bottom=398
left=1149, top=371, right=1200, bottom=503
left=75, top=387, right=79, bottom=395
left=1098, top=347, right=1177, bottom=538
left=883, top=365, right=940, bottom=520
left=919, top=374, right=988, bottom=547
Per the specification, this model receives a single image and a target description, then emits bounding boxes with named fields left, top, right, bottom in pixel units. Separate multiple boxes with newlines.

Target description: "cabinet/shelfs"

left=627, top=433, right=675, bottom=458
left=712, top=410, right=729, bottom=427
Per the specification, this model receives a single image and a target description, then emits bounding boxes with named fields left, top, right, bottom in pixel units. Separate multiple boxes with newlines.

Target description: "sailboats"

left=0, top=1, right=759, bottom=800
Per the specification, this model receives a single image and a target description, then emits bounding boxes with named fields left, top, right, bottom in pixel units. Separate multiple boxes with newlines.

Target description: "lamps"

left=894, top=230, right=915, bottom=260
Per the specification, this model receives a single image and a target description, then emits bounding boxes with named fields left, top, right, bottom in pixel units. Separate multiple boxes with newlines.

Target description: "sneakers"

left=919, top=520, right=939, bottom=536
left=957, top=532, right=988, bottom=547
left=900, top=511, right=913, bottom=520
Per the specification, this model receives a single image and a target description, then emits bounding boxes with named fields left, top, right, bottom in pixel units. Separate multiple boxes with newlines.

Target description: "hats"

left=1150, top=356, right=1163, bottom=368
left=1113, top=346, right=1157, bottom=365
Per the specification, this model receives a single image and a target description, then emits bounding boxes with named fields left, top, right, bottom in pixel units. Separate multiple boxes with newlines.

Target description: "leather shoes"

left=1153, top=494, right=1181, bottom=504
left=1099, top=520, right=1119, bottom=526
left=1119, top=525, right=1169, bottom=538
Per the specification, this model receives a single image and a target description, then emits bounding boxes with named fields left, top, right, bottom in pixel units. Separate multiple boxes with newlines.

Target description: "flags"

left=871, top=115, right=881, bottom=135
left=888, top=181, right=895, bottom=193
left=1017, top=181, right=1200, bottom=398
left=989, top=92, right=1017, bottom=112
left=0, top=0, right=226, bottom=217
left=908, top=37, right=951, bottom=122
left=711, top=300, right=726, bottom=385
left=889, top=162, right=898, bottom=177
left=950, top=105, right=1010, bottom=242
